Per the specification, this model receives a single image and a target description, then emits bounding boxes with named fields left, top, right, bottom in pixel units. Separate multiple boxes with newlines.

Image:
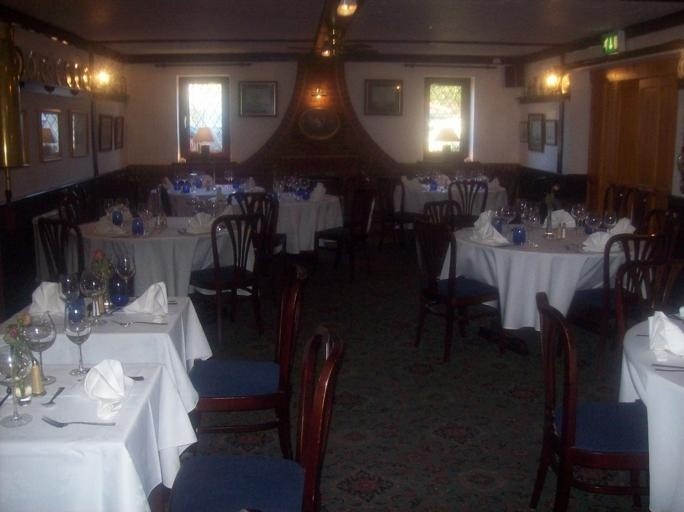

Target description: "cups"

left=103, top=197, right=166, bottom=238
left=490, top=200, right=616, bottom=248
left=430, top=182, right=436, bottom=191
left=271, top=175, right=310, bottom=200
left=173, top=167, right=255, bottom=192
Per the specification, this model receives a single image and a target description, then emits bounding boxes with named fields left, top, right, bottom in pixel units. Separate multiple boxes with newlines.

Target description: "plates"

left=15, top=47, right=92, bottom=92
left=180, top=228, right=211, bottom=236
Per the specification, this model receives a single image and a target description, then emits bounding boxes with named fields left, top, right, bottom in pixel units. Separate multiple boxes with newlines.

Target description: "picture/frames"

left=36, top=107, right=63, bottom=162
left=239, top=80, right=278, bottom=118
left=518, top=111, right=557, bottom=154
left=364, top=77, right=402, bottom=116
left=114, top=116, right=124, bottom=148
left=68, top=109, right=92, bottom=160
left=96, top=112, right=114, bottom=151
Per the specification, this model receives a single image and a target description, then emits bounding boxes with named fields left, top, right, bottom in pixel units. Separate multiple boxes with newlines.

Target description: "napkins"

left=649, top=311, right=684, bottom=361
left=123, top=281, right=168, bottom=317
left=27, top=281, right=73, bottom=316
left=84, top=360, right=135, bottom=420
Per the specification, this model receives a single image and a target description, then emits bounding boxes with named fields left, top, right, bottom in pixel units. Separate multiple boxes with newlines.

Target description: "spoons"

left=42, top=385, right=64, bottom=408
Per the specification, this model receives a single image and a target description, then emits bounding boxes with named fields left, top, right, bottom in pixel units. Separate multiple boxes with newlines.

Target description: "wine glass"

left=21, top=312, right=56, bottom=385
left=59, top=251, right=137, bottom=375
left=0, top=344, right=32, bottom=428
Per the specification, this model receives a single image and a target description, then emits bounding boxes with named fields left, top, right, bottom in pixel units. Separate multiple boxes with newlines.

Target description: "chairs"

left=188, top=280, right=303, bottom=461
left=615, top=259, right=683, bottom=353
left=169, top=323, right=347, bottom=512
left=529, top=292, right=650, bottom=512
left=0, top=171, right=405, bottom=345
left=392, top=166, right=684, bottom=363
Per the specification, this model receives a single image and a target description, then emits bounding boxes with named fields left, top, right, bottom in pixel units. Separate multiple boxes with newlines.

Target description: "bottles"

left=29, top=359, right=47, bottom=396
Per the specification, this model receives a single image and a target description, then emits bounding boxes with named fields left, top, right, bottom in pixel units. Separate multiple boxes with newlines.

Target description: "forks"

left=112, top=319, right=168, bottom=327
left=41, top=415, right=116, bottom=428
